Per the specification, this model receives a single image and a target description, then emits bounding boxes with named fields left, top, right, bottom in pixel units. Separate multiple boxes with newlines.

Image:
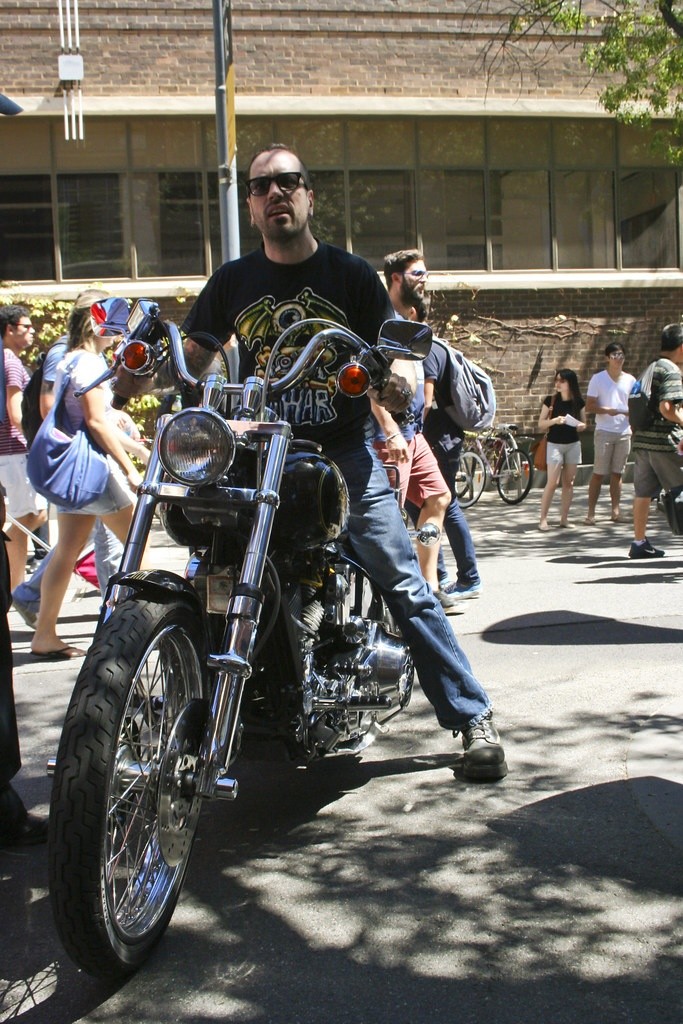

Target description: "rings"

left=110, top=376, right=118, bottom=395
left=401, top=387, right=411, bottom=400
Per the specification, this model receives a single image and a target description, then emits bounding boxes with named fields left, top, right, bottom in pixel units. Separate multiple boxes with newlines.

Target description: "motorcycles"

left=46, top=299, right=432, bottom=984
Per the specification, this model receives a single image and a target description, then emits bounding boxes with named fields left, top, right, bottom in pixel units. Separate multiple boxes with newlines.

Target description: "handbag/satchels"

left=26, top=353, right=109, bottom=510
left=532, top=437, right=547, bottom=470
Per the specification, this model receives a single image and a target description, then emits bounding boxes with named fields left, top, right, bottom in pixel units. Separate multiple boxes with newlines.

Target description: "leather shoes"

left=462, top=718, right=508, bottom=779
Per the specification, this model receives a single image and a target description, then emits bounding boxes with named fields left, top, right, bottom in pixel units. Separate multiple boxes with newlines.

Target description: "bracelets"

left=385, top=432, right=402, bottom=441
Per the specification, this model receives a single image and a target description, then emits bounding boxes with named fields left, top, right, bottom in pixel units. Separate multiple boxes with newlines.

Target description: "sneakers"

left=442, top=582, right=479, bottom=600
left=629, top=537, right=664, bottom=558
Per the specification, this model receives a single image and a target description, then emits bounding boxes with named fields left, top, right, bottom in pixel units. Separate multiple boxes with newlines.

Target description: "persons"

left=585, top=342, right=638, bottom=525
left=0, top=483, right=51, bottom=849
left=538, top=368, right=587, bottom=531
left=110, top=145, right=506, bottom=781
left=368, top=249, right=481, bottom=609
left=0, top=290, right=151, bottom=659
left=628, top=323, right=683, bottom=559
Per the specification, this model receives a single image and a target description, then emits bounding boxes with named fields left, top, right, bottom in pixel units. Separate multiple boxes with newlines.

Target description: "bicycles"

left=454, top=452, right=486, bottom=509
left=455, top=422, right=533, bottom=505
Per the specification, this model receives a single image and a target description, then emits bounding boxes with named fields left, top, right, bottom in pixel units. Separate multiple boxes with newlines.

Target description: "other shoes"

left=12, top=599, right=38, bottom=629
left=612, top=516, right=628, bottom=523
left=27, top=555, right=38, bottom=565
left=560, top=521, right=575, bottom=528
left=8, top=814, right=49, bottom=845
left=434, top=592, right=457, bottom=607
left=538, top=525, right=550, bottom=531
left=584, top=518, right=595, bottom=525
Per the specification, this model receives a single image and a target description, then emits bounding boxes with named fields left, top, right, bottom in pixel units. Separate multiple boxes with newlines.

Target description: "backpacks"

left=432, top=337, right=497, bottom=432
left=628, top=361, right=657, bottom=430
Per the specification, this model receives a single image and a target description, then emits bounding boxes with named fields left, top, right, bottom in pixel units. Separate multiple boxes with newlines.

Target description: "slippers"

left=30, top=647, right=86, bottom=658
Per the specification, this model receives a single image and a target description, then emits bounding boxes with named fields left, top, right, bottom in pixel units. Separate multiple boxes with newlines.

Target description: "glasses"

left=555, top=378, right=566, bottom=383
left=17, top=324, right=34, bottom=331
left=610, top=353, right=624, bottom=359
left=245, top=172, right=309, bottom=196
left=404, top=271, right=428, bottom=279
